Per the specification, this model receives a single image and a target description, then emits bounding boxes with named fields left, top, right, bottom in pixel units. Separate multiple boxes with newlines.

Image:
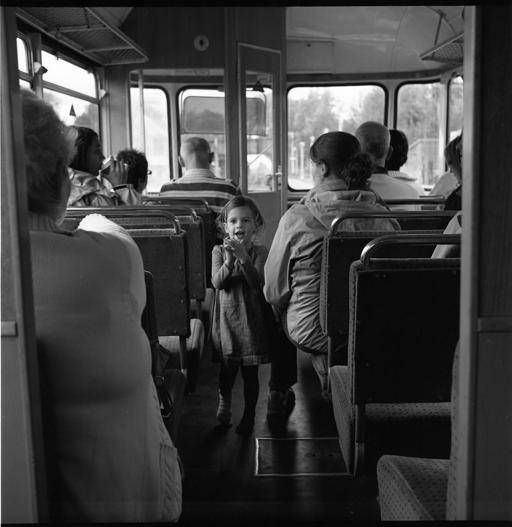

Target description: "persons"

left=23, top=88, right=183, bottom=526
left=442, top=137, right=463, bottom=213
left=389, top=129, right=425, bottom=197
left=66, top=126, right=143, bottom=207
left=211, top=196, right=280, bottom=436
left=263, top=132, right=401, bottom=428
left=355, top=122, right=422, bottom=213
left=116, top=149, right=162, bottom=206
left=160, top=137, right=242, bottom=215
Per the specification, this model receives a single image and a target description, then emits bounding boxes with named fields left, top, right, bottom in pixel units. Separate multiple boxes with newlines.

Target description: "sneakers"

left=266, top=388, right=295, bottom=428
left=217, top=394, right=232, bottom=424
left=234, top=410, right=255, bottom=437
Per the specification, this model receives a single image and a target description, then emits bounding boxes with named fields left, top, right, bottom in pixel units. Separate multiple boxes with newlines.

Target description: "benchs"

left=309, top=210, right=465, bottom=400
left=375, top=333, right=465, bottom=521
left=367, top=198, right=448, bottom=206
left=143, top=196, right=218, bottom=291
left=139, top=270, right=187, bottom=451
left=326, top=233, right=461, bottom=480
left=62, top=210, right=207, bottom=389
left=67, top=205, right=216, bottom=345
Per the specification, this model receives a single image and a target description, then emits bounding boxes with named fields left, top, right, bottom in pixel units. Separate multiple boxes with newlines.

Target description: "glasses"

left=146, top=170, right=153, bottom=175
